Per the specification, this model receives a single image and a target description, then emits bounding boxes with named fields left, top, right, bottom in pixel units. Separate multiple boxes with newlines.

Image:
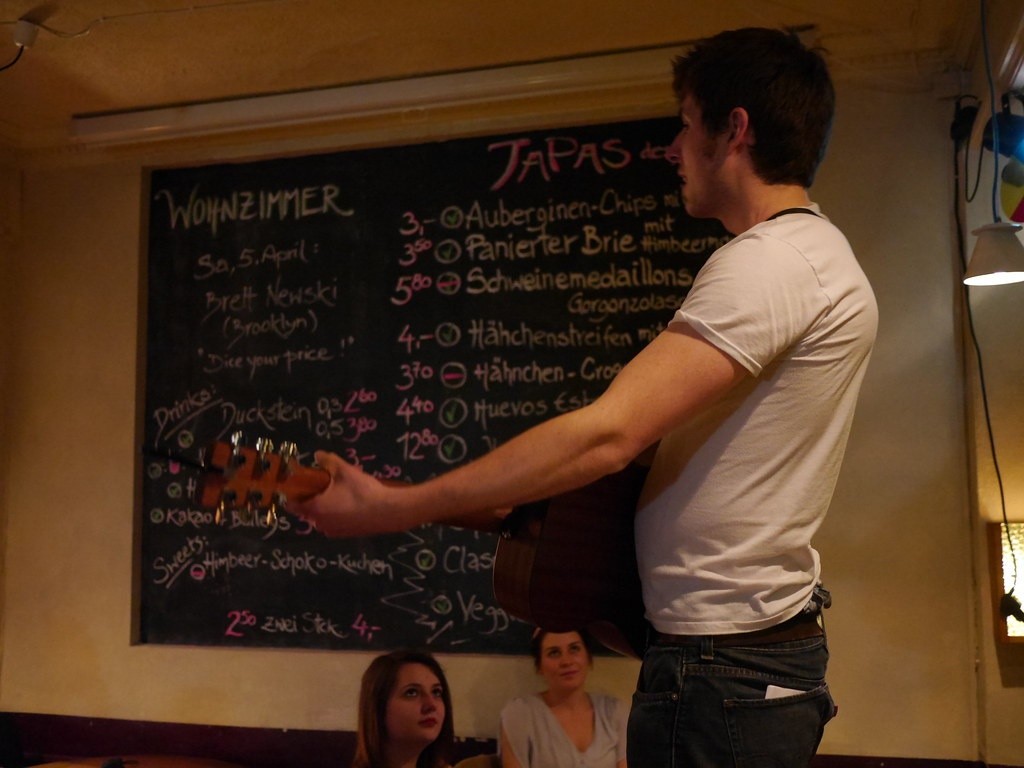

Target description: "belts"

left=651, top=610, right=824, bottom=649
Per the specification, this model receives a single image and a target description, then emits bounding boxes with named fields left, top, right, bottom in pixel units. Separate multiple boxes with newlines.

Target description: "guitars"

left=195, top=428, right=647, bottom=659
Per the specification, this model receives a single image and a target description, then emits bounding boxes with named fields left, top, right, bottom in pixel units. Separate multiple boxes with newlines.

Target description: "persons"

left=498, top=624, right=629, bottom=767
left=279, top=26, right=879, bottom=768
left=351, top=650, right=456, bottom=768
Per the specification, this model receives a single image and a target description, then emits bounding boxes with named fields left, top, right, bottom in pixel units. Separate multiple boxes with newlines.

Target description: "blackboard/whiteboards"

left=130, top=107, right=737, bottom=659
